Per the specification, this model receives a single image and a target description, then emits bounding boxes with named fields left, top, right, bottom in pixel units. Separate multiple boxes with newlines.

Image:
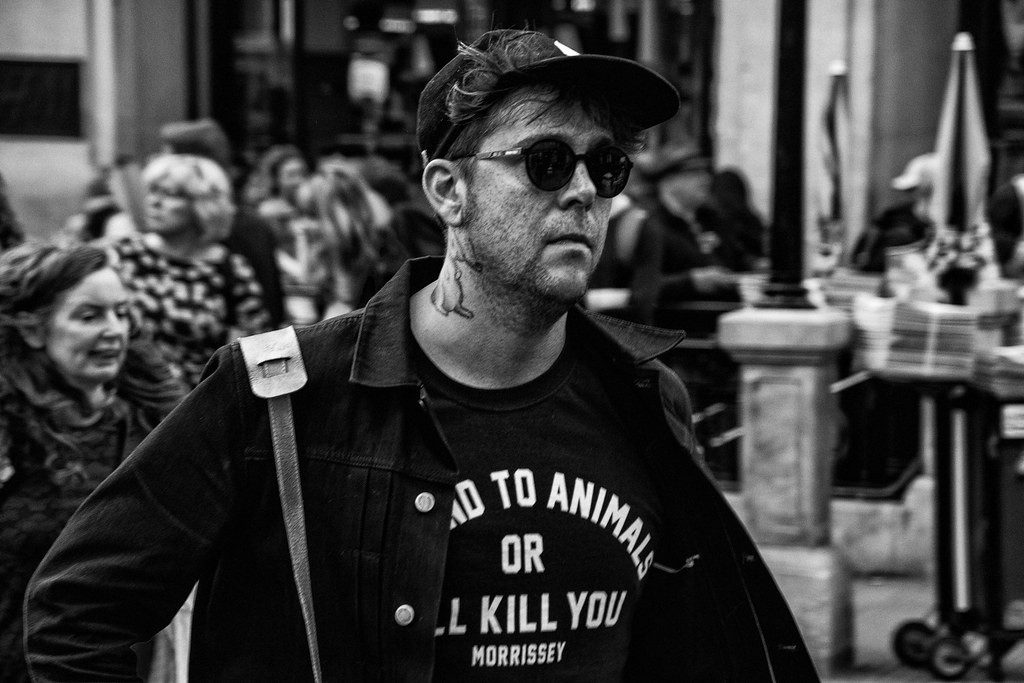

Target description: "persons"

left=589, top=164, right=1024, bottom=310
left=0, top=245, right=186, bottom=683
left=23, top=32, right=820, bottom=683
left=66, top=119, right=425, bottom=395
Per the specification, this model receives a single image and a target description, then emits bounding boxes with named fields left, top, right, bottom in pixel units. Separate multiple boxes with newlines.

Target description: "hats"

left=417, top=27, right=682, bottom=167
left=891, top=152, right=935, bottom=191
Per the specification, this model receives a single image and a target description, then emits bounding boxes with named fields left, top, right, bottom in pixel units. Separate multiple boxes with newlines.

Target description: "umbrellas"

left=929, top=32, right=994, bottom=604
left=815, top=61, right=852, bottom=264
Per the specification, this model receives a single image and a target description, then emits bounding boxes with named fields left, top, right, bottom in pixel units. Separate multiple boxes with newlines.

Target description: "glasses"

left=451, top=137, right=636, bottom=199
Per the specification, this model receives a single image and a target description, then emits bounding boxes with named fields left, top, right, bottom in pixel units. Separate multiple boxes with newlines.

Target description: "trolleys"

left=893, top=374, right=1024, bottom=681
